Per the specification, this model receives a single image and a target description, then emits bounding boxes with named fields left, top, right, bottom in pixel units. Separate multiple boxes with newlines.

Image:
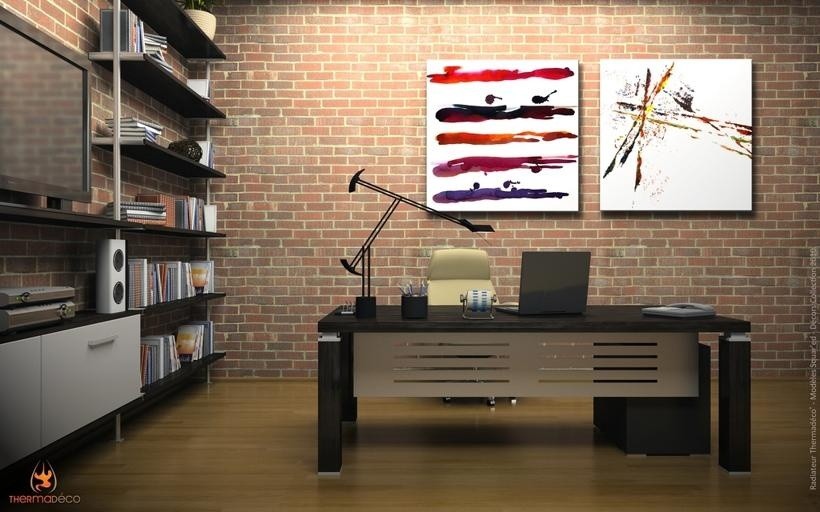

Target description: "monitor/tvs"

left=0, top=6, right=94, bottom=203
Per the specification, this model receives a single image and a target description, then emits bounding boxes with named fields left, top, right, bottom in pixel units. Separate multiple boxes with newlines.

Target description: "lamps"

left=340, top=170, right=495, bottom=320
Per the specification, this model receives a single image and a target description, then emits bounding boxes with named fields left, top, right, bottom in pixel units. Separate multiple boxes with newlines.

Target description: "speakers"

left=96, top=238, right=127, bottom=314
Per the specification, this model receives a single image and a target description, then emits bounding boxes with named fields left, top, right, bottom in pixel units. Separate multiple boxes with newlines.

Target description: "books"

left=94, top=9, right=215, bottom=389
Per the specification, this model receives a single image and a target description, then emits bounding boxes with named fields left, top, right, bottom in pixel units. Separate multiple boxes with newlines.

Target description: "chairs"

left=426, top=247, right=522, bottom=407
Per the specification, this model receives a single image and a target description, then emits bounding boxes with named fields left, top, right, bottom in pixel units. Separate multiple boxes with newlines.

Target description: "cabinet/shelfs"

left=593, top=344, right=712, bottom=454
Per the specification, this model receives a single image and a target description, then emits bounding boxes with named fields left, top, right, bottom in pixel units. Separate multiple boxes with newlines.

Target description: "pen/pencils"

left=397, top=279, right=429, bottom=296
left=334, top=311, right=355, bottom=315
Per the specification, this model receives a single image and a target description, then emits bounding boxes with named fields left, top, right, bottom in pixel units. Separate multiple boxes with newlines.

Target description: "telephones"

left=642, top=302, right=716, bottom=317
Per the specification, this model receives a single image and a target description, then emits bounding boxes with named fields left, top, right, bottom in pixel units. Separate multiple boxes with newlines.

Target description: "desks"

left=318, top=305, right=752, bottom=479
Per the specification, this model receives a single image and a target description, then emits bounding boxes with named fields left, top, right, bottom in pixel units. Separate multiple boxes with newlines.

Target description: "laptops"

left=495, top=250, right=592, bottom=315
